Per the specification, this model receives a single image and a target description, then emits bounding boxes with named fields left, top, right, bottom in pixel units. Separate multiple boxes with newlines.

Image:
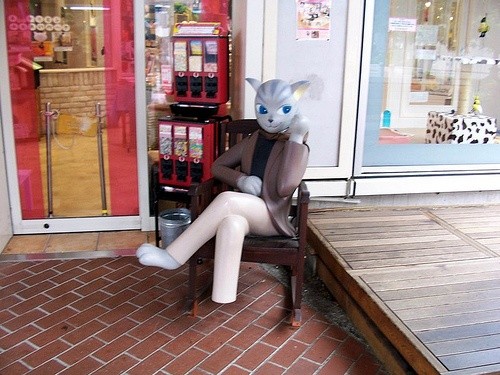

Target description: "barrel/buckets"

left=159, top=208, right=191, bottom=249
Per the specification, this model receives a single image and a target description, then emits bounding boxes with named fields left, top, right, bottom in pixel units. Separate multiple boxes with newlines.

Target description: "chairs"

left=189, top=120, right=311, bottom=328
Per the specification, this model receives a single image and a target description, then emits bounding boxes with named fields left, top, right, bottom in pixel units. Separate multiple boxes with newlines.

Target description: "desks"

left=425, top=112, right=497, bottom=144
